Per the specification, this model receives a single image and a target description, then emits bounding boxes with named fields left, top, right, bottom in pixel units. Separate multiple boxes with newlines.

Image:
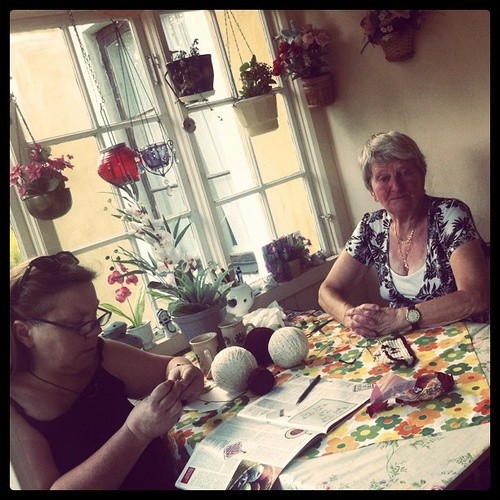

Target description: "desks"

left=128, top=310, right=490, bottom=490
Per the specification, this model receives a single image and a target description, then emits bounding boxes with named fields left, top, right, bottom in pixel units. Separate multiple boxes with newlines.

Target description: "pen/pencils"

left=295, top=374, right=321, bottom=406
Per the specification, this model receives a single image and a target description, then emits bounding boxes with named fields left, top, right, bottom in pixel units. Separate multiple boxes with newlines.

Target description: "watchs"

left=405, top=303, right=421, bottom=330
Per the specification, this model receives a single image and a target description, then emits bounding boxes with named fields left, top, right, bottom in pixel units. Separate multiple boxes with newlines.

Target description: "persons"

left=317, top=128, right=491, bottom=337
left=9, top=251, right=203, bottom=490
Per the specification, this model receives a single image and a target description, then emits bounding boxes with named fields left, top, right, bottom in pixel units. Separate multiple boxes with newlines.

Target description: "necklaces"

left=393, top=216, right=421, bottom=274
left=28, top=366, right=100, bottom=399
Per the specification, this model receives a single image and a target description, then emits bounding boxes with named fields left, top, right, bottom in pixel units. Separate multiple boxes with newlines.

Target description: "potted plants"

left=166, top=38, right=214, bottom=102
left=232, top=53, right=279, bottom=138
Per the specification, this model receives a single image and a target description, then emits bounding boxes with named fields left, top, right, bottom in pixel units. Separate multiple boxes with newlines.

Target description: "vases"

left=287, top=259, right=301, bottom=278
left=21, top=187, right=72, bottom=220
left=171, top=300, right=227, bottom=349
left=125, top=320, right=155, bottom=350
left=301, top=74, right=334, bottom=108
left=381, top=32, right=415, bottom=62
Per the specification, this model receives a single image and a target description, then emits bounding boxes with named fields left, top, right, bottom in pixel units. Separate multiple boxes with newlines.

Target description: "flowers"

left=267, top=22, right=336, bottom=80
left=358, top=10, right=421, bottom=55
left=96, top=181, right=231, bottom=326
left=266, top=234, right=312, bottom=262
left=10, top=144, right=74, bottom=197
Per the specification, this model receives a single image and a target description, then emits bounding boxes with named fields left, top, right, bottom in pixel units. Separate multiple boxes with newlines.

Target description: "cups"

left=143, top=141, right=170, bottom=170
left=217, top=316, right=256, bottom=348
left=189, top=331, right=219, bottom=379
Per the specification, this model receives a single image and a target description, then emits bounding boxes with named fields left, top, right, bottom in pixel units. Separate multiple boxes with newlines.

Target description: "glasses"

left=304, top=334, right=368, bottom=364
left=12, top=251, right=80, bottom=299
left=31, top=306, right=112, bottom=335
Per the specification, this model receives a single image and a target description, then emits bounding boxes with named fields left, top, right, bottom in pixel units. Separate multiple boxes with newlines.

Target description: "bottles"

left=98, top=142, right=141, bottom=185
left=222, top=283, right=254, bottom=318
